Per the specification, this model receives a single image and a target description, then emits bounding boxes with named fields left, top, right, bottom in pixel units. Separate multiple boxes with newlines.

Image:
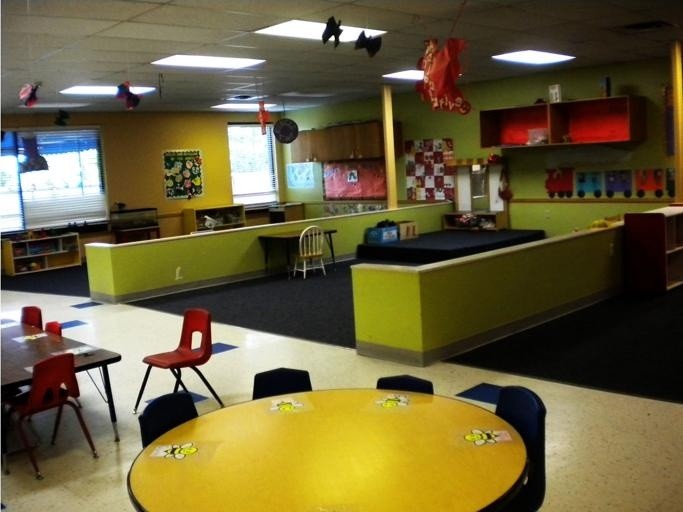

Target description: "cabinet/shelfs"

left=290, top=130, right=313, bottom=164
left=443, top=211, right=505, bottom=232
left=2, top=232, right=82, bottom=278
left=182, top=205, right=247, bottom=234
left=478, top=95, right=646, bottom=149
left=269, top=204, right=306, bottom=223
left=624, top=204, right=683, bottom=291
left=343, top=121, right=400, bottom=160
left=310, top=126, right=344, bottom=162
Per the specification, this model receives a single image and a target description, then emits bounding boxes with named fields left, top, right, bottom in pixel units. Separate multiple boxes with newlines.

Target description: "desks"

left=127, top=388, right=533, bottom=512
left=258, top=228, right=336, bottom=270
left=2, top=320, right=122, bottom=442
left=115, top=226, right=161, bottom=243
left=356, top=228, right=545, bottom=267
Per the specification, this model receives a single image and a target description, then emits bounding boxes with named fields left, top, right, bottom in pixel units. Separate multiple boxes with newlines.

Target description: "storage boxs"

left=366, top=226, right=399, bottom=244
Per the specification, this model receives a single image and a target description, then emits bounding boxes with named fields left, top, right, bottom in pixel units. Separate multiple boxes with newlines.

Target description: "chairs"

left=135, top=308, right=312, bottom=449
left=22, top=305, right=63, bottom=334
left=487, top=387, right=547, bottom=512
left=293, top=225, right=327, bottom=279
left=7, top=354, right=99, bottom=478
left=377, top=374, right=434, bottom=393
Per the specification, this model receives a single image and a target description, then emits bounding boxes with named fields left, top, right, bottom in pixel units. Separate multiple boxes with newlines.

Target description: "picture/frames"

left=160, top=149, right=205, bottom=200
